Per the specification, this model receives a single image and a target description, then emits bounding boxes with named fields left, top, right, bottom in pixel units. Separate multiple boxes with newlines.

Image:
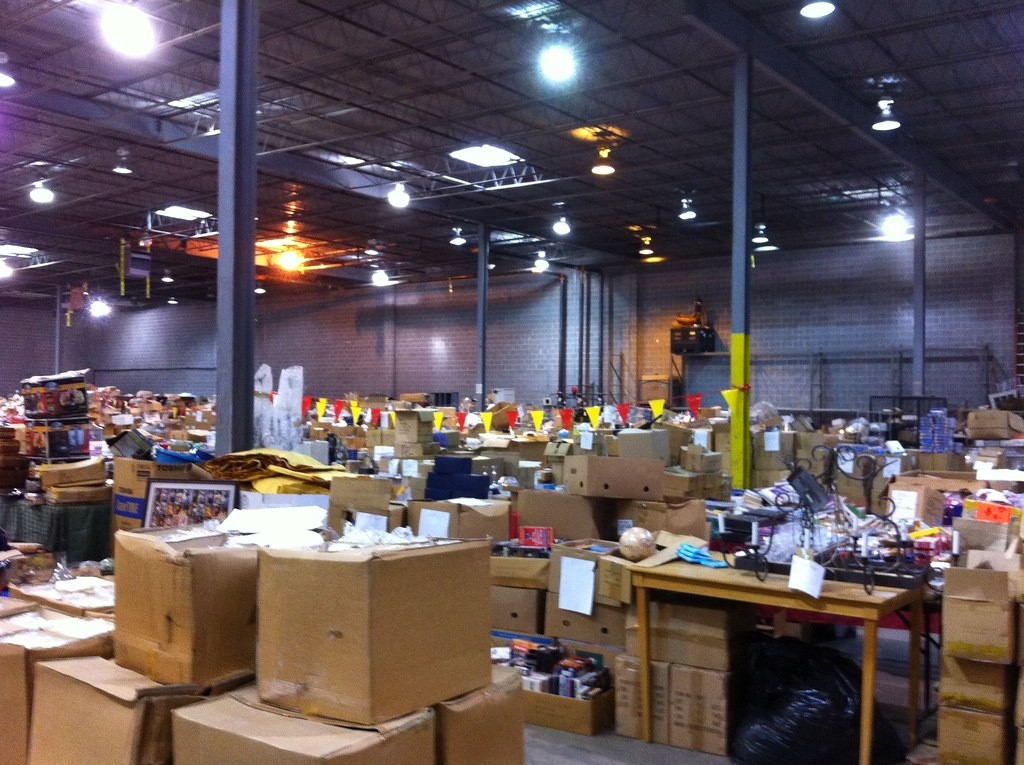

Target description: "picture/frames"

left=140, top=477, right=238, bottom=528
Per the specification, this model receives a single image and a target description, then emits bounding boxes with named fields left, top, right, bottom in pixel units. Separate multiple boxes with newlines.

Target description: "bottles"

left=324, top=433, right=337, bottom=465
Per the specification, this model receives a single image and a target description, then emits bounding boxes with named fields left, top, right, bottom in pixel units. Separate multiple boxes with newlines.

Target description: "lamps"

left=448, top=229, right=466, bottom=245
left=750, top=222, right=768, bottom=243
left=161, top=271, right=174, bottom=282
left=589, top=143, right=615, bottom=175
left=870, top=95, right=900, bottom=131
left=676, top=194, right=697, bottom=220
left=110, top=147, right=132, bottom=174
left=29, top=182, right=54, bottom=202
left=639, top=236, right=653, bottom=254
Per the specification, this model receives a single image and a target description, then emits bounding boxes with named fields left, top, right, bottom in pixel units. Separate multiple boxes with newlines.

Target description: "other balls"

left=619, top=527, right=656, bottom=562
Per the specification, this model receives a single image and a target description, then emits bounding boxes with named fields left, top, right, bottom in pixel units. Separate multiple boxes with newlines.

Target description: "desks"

left=624, top=551, right=924, bottom=765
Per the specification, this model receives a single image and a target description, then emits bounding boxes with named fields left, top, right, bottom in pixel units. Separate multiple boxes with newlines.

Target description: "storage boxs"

left=0, top=380, right=1024, bottom=765
